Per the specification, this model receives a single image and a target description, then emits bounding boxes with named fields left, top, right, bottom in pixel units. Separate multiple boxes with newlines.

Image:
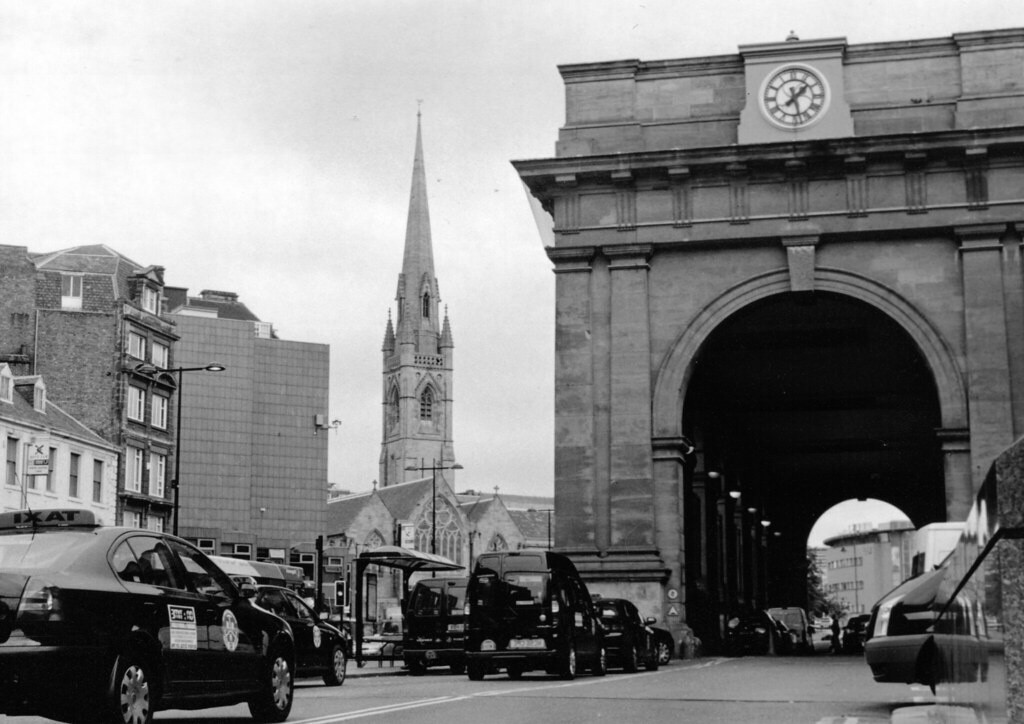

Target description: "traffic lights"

left=334, top=581, right=347, bottom=606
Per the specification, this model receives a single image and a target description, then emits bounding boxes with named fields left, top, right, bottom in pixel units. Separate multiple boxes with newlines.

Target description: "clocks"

left=758, top=63, right=832, bottom=131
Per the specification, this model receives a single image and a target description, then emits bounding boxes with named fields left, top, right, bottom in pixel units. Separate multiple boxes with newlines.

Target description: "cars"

left=352, top=634, right=393, bottom=659
left=842, top=615, right=871, bottom=653
left=725, top=611, right=798, bottom=656
left=591, top=597, right=674, bottom=674
left=0, top=522, right=296, bottom=724
left=864, top=544, right=956, bottom=684
left=236, top=583, right=352, bottom=687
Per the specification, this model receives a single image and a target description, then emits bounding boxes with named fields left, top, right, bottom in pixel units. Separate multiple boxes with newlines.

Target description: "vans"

left=768, top=607, right=816, bottom=651
left=465, top=551, right=607, bottom=680
left=400, top=578, right=467, bottom=676
left=152, top=552, right=315, bottom=607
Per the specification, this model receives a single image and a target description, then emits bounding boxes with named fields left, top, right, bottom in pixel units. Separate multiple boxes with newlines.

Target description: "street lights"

left=527, top=508, right=555, bottom=551
left=135, top=362, right=225, bottom=536
left=405, top=462, right=463, bottom=577
